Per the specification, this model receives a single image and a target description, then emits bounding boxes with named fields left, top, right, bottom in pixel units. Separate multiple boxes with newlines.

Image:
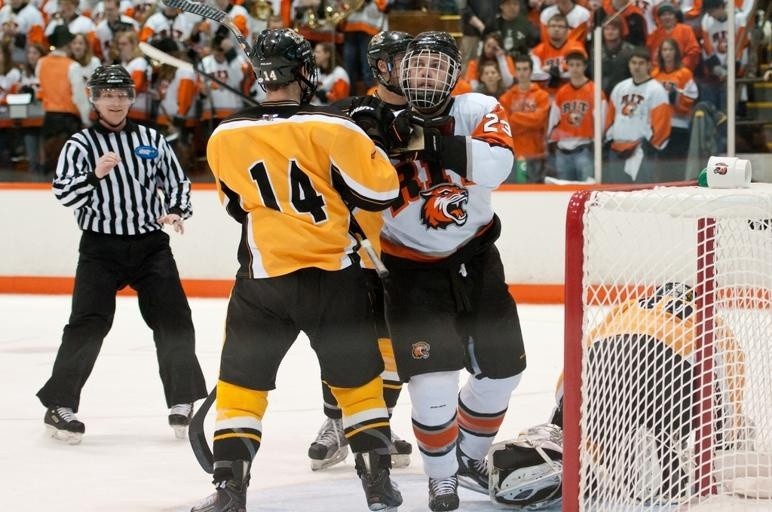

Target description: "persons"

left=459, top=2, right=487, bottom=41
left=46, top=0, right=101, bottom=45
left=34, top=63, right=208, bottom=445
left=4, top=45, right=45, bottom=173
left=531, top=14, right=588, bottom=72
left=141, top=3, right=190, bottom=54
left=369, top=28, right=528, bottom=511
left=310, top=42, right=351, bottom=108
left=98, top=0, right=142, bottom=56
left=499, top=55, right=550, bottom=185
left=215, top=0, right=253, bottom=53
left=342, top=0, right=391, bottom=94
left=69, top=32, right=102, bottom=81
left=702, top=0, right=746, bottom=116
left=190, top=27, right=405, bottom=511
left=196, top=35, right=254, bottom=124
left=34, top=25, right=93, bottom=173
left=307, top=27, right=419, bottom=473
left=652, top=39, right=698, bottom=150
left=649, top=5, right=700, bottom=78
left=546, top=44, right=615, bottom=186
left=153, top=38, right=196, bottom=146
left=482, top=0, right=538, bottom=48
left=0, top=0, right=46, bottom=64
left=471, top=60, right=508, bottom=99
left=464, top=32, right=515, bottom=83
left=538, top=0, right=592, bottom=40
left=116, top=32, right=153, bottom=128
left=605, top=47, right=671, bottom=185
left=486, top=273, right=745, bottom=512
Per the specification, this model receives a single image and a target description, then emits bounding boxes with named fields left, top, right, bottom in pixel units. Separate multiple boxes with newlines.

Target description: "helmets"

left=250, top=29, right=319, bottom=105
left=89, top=64, right=139, bottom=105
left=366, top=31, right=415, bottom=96
left=398, top=31, right=462, bottom=114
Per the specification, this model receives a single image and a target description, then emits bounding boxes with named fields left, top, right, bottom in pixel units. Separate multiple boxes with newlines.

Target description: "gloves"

left=349, top=97, right=388, bottom=150
left=388, top=110, right=455, bottom=160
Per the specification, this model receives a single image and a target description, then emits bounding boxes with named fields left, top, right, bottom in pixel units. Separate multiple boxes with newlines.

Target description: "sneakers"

left=168, top=402, right=193, bottom=425
left=309, top=422, right=350, bottom=460
left=390, top=431, right=411, bottom=455
left=190, top=486, right=246, bottom=511
left=428, top=477, right=459, bottom=512
left=455, top=449, right=489, bottom=488
left=44, top=405, right=85, bottom=433
left=362, top=472, right=403, bottom=510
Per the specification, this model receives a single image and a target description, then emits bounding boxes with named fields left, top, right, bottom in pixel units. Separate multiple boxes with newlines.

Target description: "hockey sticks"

left=163, top=1, right=391, bottom=280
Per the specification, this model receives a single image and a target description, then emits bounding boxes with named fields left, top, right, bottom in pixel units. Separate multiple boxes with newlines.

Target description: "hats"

left=656, top=1, right=680, bottom=14
left=47, top=25, right=76, bottom=48
